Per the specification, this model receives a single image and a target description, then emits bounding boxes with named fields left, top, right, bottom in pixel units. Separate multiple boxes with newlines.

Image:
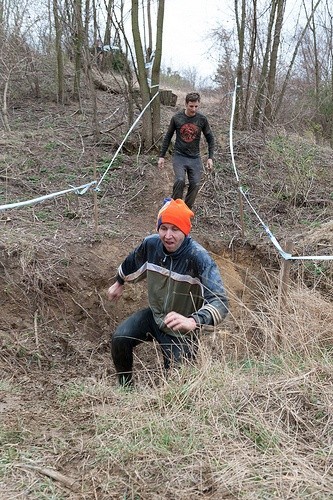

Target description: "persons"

left=105, top=198, right=230, bottom=392
left=157, top=90, right=217, bottom=212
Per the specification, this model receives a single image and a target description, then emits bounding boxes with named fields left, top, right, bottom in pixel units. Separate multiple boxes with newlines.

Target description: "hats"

left=158, top=198, right=194, bottom=236
